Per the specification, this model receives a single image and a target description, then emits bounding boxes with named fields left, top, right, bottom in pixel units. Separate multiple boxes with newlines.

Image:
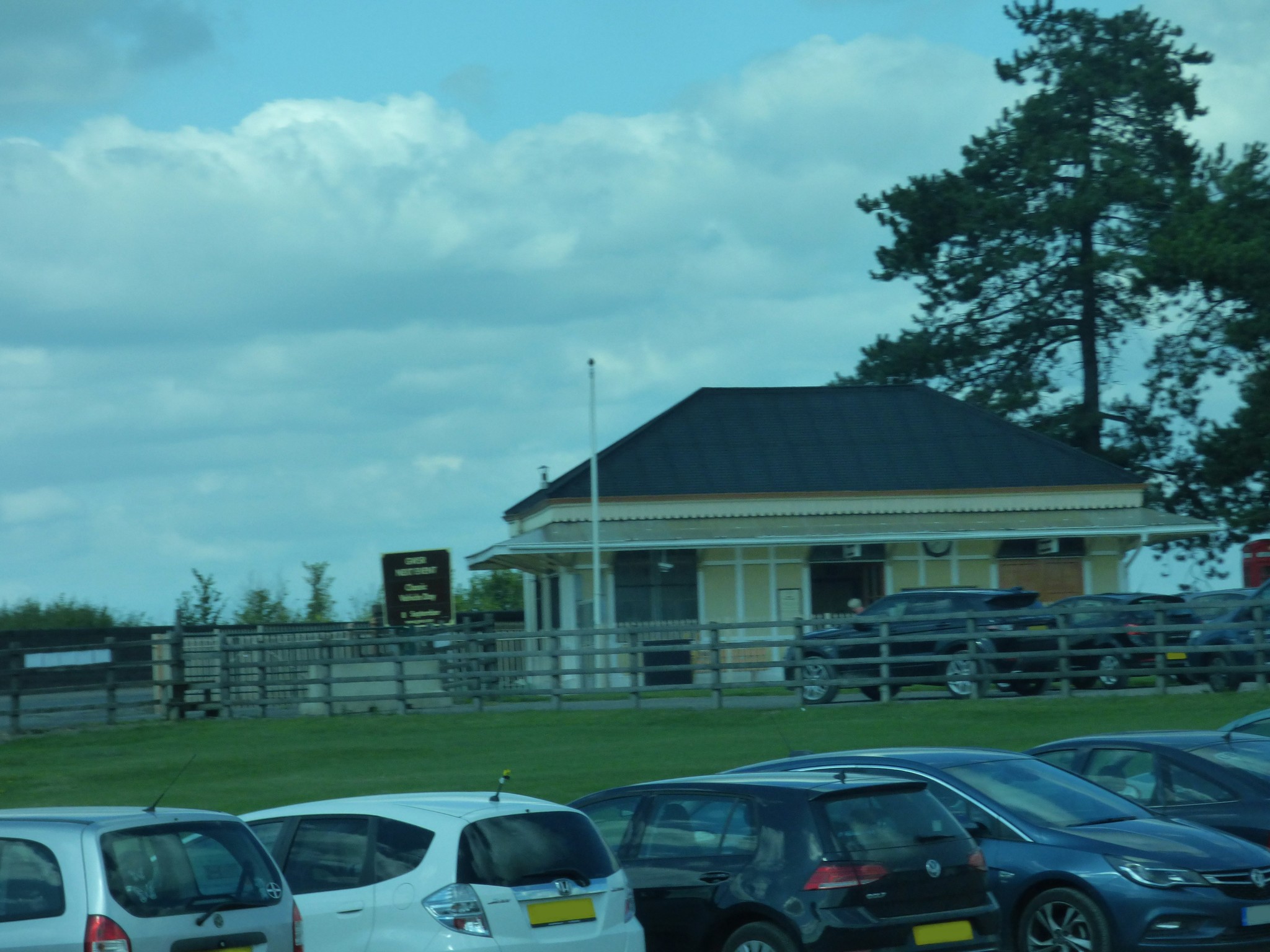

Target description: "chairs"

left=646, top=804, right=700, bottom=869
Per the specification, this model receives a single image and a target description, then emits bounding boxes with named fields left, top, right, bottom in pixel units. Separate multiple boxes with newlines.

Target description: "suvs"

left=783, top=584, right=1064, bottom=706
left=1040, top=591, right=1206, bottom=691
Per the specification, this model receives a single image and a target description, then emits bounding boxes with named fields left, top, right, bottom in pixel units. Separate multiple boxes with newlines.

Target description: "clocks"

left=924, top=540, right=951, bottom=557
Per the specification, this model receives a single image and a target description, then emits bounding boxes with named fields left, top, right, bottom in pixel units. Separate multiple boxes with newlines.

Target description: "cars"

left=642, top=745, right=1269, bottom=952
left=0, top=803, right=305, bottom=952
left=1165, top=573, right=1270, bottom=692
left=1215, top=708, right=1270, bottom=739
left=536, top=768, right=1004, bottom=952
left=1018, top=728, right=1270, bottom=849
left=123, top=789, right=648, bottom=952
